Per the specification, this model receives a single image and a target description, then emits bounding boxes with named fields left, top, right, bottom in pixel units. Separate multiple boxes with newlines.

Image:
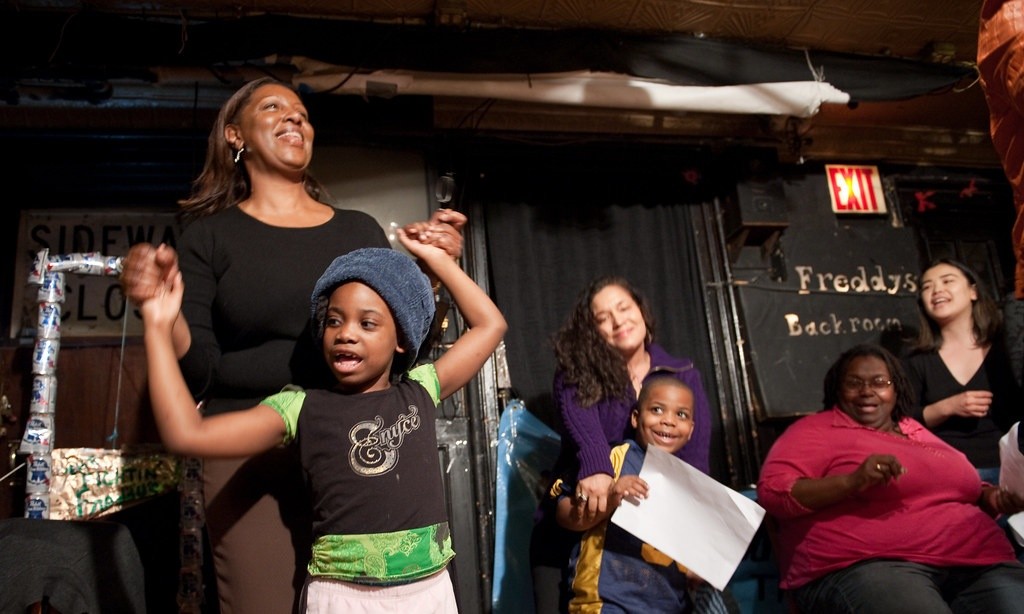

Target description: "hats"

left=310, top=247, right=434, bottom=368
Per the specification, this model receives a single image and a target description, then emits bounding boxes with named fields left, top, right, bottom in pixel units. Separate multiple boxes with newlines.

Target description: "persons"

left=897, top=260, right=1024, bottom=563
left=756, top=343, right=1023, bottom=614
left=548, top=368, right=696, bottom=612
left=122, top=220, right=510, bottom=612
left=121, top=79, right=468, bottom=612
left=529, top=272, right=713, bottom=614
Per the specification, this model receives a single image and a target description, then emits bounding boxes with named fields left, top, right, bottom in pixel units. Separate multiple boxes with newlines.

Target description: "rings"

left=576, top=494, right=587, bottom=502
left=876, top=463, right=881, bottom=470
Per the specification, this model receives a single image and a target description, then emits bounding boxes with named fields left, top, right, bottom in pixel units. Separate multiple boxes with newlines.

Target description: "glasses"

left=843, top=375, right=893, bottom=389
